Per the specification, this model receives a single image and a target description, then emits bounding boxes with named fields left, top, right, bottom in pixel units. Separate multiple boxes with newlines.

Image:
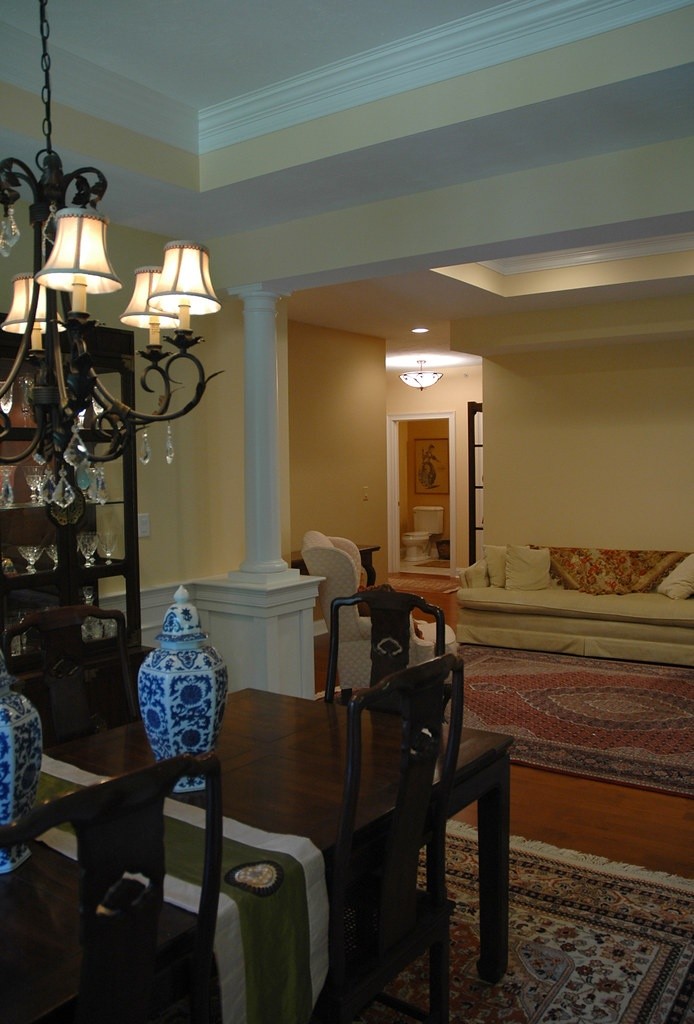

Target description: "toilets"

left=401, top=505, right=444, bottom=562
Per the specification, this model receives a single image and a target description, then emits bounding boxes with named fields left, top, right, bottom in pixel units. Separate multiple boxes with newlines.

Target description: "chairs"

left=307, top=654, right=464, bottom=1024
left=0, top=604, right=141, bottom=742
left=325, top=587, right=446, bottom=715
left=302, top=531, right=457, bottom=689
left=0, top=750, right=225, bottom=1024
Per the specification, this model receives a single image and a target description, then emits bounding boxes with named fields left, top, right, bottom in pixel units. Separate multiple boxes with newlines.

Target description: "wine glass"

left=0, top=372, right=118, bottom=657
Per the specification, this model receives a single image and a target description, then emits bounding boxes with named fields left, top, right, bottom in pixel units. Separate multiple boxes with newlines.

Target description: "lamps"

left=0, top=0, right=222, bottom=510
left=399, top=360, right=444, bottom=391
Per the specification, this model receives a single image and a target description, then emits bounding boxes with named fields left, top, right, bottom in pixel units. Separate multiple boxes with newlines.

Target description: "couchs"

left=456, top=544, right=694, bottom=668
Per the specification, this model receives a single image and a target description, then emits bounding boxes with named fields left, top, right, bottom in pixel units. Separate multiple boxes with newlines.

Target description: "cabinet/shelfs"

left=0, top=310, right=157, bottom=748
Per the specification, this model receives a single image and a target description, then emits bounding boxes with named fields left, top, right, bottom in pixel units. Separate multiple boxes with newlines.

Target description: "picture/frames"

left=413, top=438, right=450, bottom=494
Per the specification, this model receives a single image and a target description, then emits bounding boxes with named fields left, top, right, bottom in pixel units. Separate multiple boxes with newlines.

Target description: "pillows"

left=482, top=545, right=530, bottom=588
left=505, top=543, right=551, bottom=591
left=358, top=584, right=423, bottom=640
left=657, top=554, right=694, bottom=601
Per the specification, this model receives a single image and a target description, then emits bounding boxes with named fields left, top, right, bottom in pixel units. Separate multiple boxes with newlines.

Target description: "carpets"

left=357, top=818, right=693, bottom=1024
left=379, top=575, right=461, bottom=593
left=441, top=644, right=694, bottom=798
left=414, top=561, right=450, bottom=568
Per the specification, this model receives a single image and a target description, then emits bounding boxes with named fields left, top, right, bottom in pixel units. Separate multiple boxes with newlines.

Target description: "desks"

left=0, top=689, right=516, bottom=1024
left=290, top=544, right=381, bottom=587
